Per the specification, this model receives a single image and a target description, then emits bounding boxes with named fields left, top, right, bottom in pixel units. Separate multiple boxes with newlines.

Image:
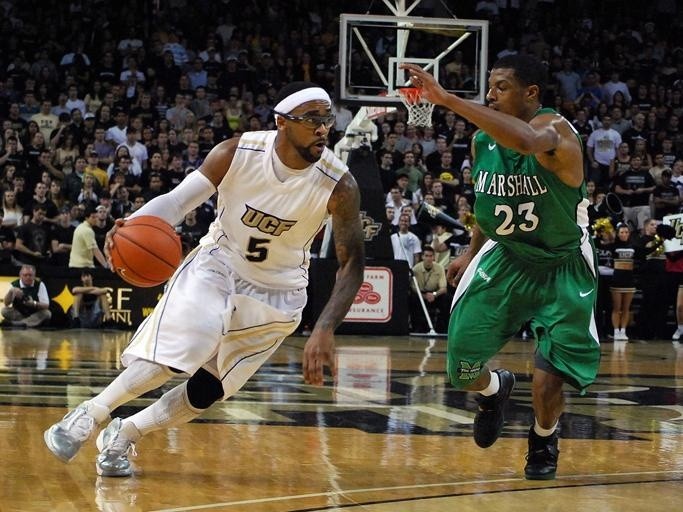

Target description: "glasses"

left=271, top=109, right=337, bottom=129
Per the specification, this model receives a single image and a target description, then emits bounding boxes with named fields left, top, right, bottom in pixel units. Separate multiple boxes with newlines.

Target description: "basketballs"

left=107, top=216, right=183, bottom=289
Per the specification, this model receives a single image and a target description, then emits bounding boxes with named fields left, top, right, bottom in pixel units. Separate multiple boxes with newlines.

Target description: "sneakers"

left=43, top=401, right=110, bottom=463
left=473, top=369, right=515, bottom=448
left=524, top=422, right=562, bottom=479
left=96, top=416, right=144, bottom=477
left=672, top=329, right=682, bottom=340
left=67, top=317, right=81, bottom=329
left=614, top=333, right=629, bottom=341
left=105, top=318, right=122, bottom=329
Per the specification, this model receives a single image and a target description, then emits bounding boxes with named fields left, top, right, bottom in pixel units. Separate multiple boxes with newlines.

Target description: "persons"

left=398, top=54, right=601, bottom=481
left=0, top=0, right=683, bottom=343
left=42, top=83, right=366, bottom=477
